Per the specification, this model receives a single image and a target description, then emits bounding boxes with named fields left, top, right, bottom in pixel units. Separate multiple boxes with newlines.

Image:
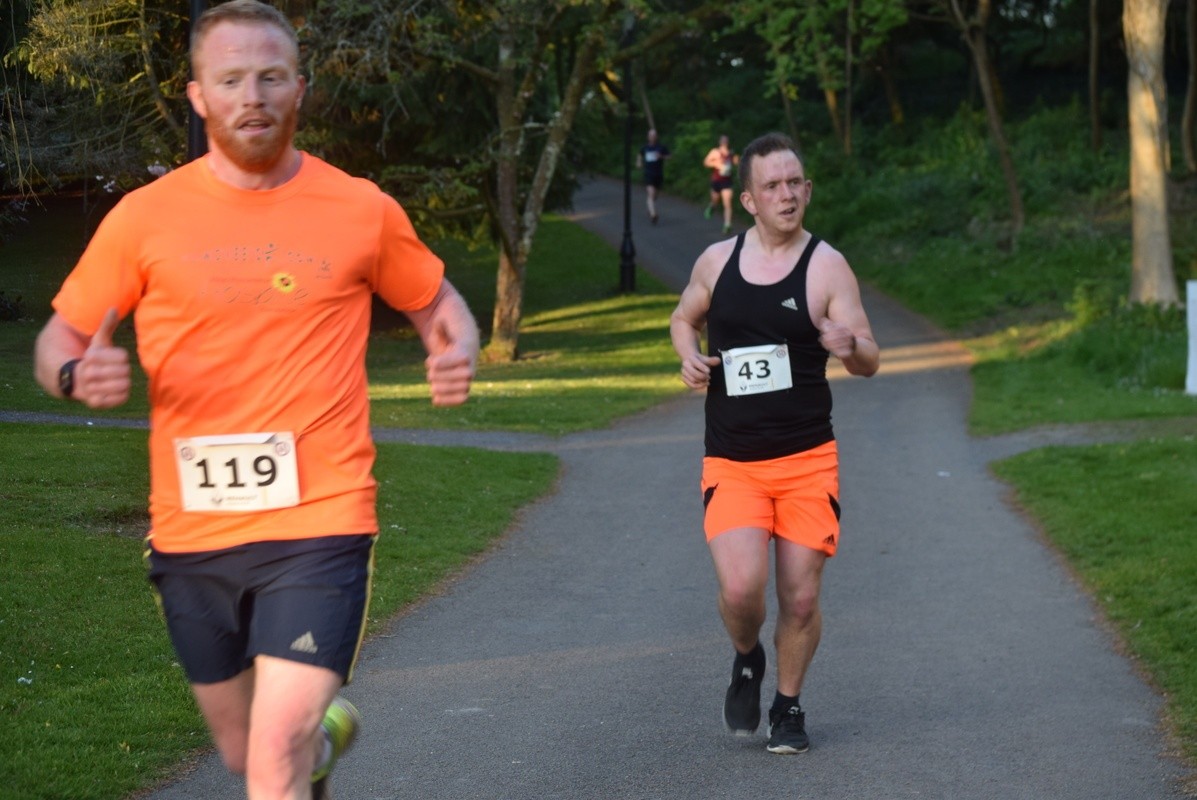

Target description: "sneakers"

left=309, top=696, right=359, bottom=800
left=723, top=643, right=766, bottom=736
left=767, top=705, right=810, bottom=753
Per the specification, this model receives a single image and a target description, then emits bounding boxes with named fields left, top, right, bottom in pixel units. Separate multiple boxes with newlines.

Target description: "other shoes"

left=652, top=213, right=660, bottom=227
left=704, top=204, right=713, bottom=220
left=723, top=225, right=731, bottom=235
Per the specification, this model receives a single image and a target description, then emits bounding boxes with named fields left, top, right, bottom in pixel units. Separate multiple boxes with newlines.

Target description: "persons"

left=668, top=134, right=881, bottom=755
left=33, top=0, right=478, bottom=800
left=703, top=136, right=740, bottom=233
left=637, top=130, right=669, bottom=222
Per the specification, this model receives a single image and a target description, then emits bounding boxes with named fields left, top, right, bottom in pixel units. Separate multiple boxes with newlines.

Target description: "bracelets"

left=850, top=333, right=856, bottom=351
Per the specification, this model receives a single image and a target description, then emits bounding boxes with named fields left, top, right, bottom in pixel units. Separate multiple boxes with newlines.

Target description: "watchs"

left=58, top=357, right=82, bottom=398
